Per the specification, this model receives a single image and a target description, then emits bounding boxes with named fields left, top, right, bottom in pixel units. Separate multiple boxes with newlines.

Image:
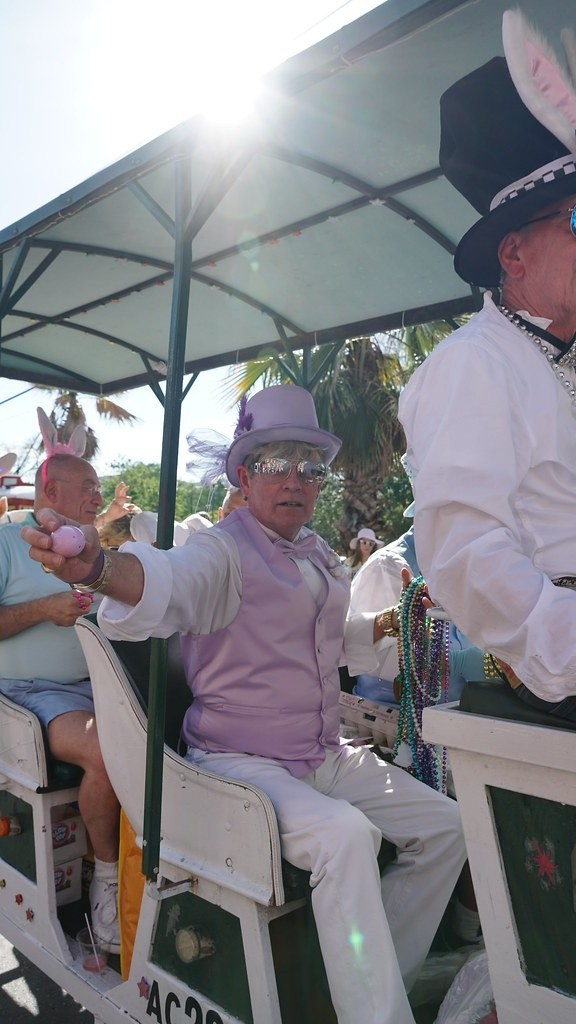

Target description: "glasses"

left=516, top=204, right=576, bottom=238
left=55, top=479, right=102, bottom=497
left=250, top=457, right=327, bottom=486
left=360, top=539, right=376, bottom=547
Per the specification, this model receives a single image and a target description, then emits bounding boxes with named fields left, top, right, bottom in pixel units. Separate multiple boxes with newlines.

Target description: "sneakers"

left=88, top=872, right=121, bottom=956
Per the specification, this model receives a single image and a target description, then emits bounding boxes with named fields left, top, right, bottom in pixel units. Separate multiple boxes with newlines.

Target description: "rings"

left=41, top=563, right=54, bottom=573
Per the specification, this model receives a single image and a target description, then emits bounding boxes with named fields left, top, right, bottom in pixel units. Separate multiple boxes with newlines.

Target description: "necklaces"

left=393, top=573, right=450, bottom=793
left=498, top=304, right=576, bottom=397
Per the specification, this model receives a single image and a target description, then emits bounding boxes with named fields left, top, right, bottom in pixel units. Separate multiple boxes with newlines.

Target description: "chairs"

left=75, top=612, right=311, bottom=1024
left=0, top=694, right=90, bottom=963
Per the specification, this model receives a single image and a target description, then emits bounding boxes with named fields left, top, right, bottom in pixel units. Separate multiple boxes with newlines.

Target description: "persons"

left=343, top=528, right=385, bottom=573
left=22, top=441, right=468, bottom=1024
left=345, top=525, right=484, bottom=746
left=393, top=194, right=576, bottom=733
left=0, top=454, right=121, bottom=955
left=95, top=481, right=250, bottom=549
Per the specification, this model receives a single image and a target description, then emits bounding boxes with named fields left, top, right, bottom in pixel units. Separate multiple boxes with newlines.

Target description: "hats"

left=349, top=528, right=384, bottom=550
left=438, top=10, right=576, bottom=289
left=225, top=385, right=342, bottom=487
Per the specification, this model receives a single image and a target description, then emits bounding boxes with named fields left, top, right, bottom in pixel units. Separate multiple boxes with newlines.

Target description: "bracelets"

left=70, top=547, right=113, bottom=595
left=483, top=652, right=504, bottom=681
left=73, top=592, right=94, bottom=610
left=382, top=607, right=399, bottom=638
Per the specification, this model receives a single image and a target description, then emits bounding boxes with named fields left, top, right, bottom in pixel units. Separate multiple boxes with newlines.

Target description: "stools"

left=420, top=679, right=576, bottom=1024
